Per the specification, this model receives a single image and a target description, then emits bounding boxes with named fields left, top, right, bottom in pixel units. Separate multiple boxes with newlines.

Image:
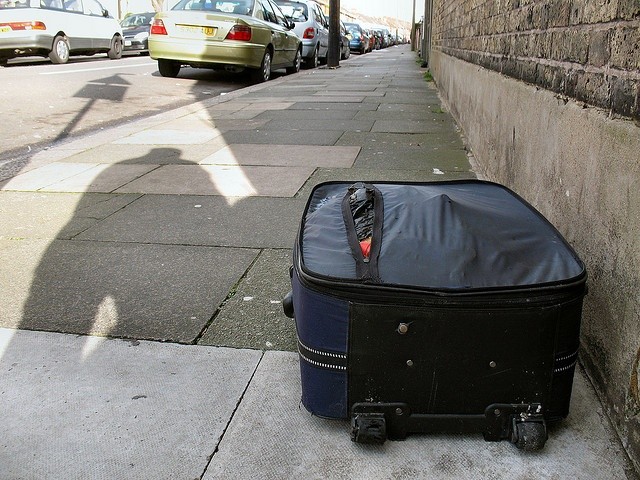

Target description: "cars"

left=343, top=23, right=370, bottom=54
left=148, top=0, right=303, bottom=82
left=119, top=12, right=157, bottom=56
left=364, top=29, right=403, bottom=50
left=318, top=15, right=350, bottom=61
left=272, top=0, right=329, bottom=68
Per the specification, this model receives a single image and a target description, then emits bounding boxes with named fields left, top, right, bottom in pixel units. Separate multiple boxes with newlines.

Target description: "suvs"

left=0, top=0, right=125, bottom=66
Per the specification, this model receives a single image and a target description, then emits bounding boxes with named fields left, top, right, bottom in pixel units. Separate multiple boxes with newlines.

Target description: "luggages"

left=281, top=178, right=590, bottom=452
